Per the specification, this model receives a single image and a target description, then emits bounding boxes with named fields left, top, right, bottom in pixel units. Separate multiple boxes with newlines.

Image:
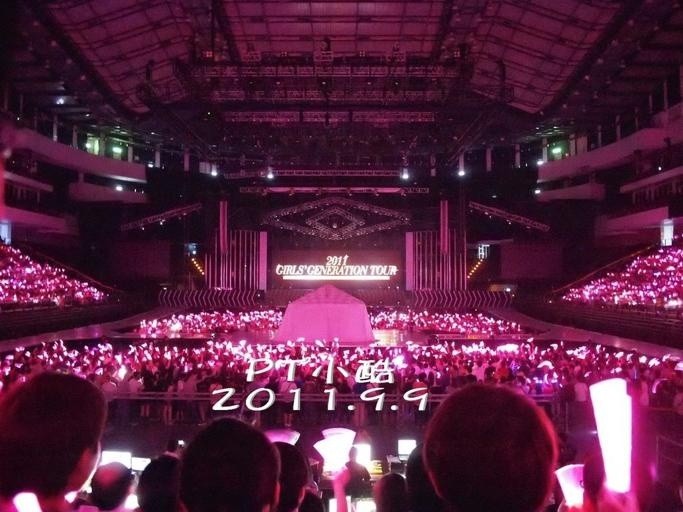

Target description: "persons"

left=1, top=227, right=680, bottom=512
